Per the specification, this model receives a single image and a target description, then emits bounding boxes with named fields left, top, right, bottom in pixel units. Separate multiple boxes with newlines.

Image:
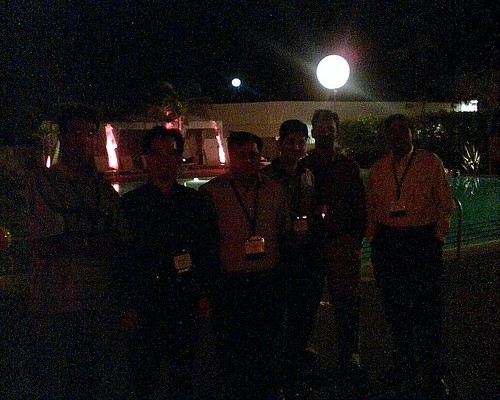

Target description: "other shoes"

left=344, top=352, right=366, bottom=380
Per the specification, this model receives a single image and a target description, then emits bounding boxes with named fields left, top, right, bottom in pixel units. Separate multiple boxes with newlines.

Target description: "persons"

left=199, top=130, right=291, bottom=400
left=296, top=109, right=367, bottom=380
left=261, top=119, right=319, bottom=363
left=122, top=125, right=221, bottom=400
left=27, top=104, right=134, bottom=399
left=367, top=114, right=456, bottom=395
